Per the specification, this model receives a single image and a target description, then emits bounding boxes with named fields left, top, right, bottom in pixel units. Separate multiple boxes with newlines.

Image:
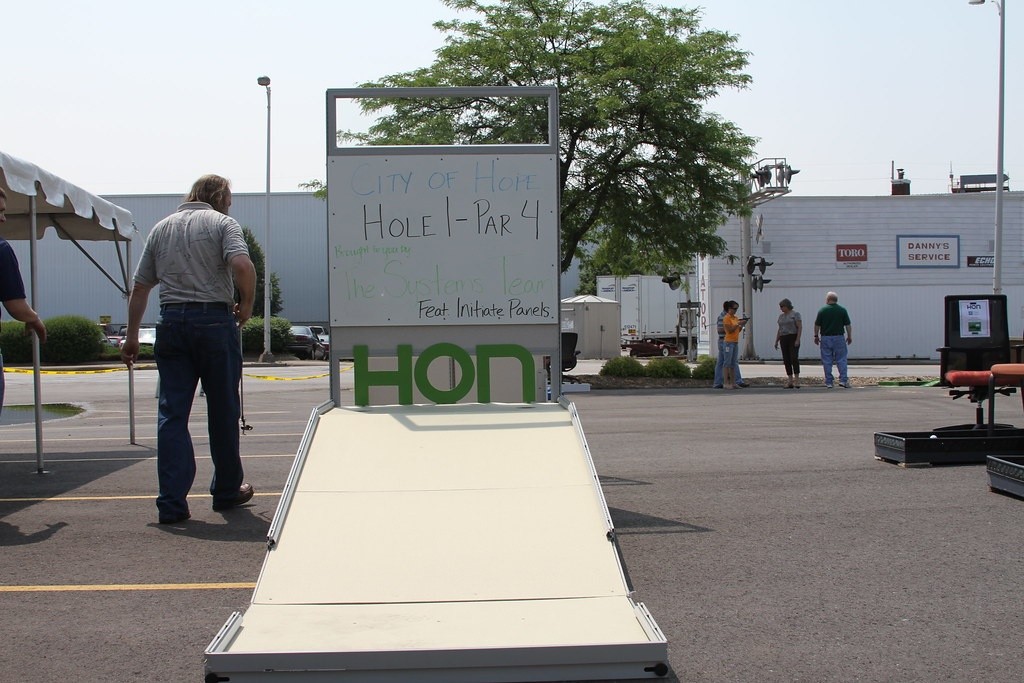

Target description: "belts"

left=821, top=333, right=843, bottom=336
left=162, top=301, right=232, bottom=311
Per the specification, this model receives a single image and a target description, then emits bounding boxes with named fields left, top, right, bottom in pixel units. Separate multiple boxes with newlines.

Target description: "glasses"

left=730, top=307, right=738, bottom=311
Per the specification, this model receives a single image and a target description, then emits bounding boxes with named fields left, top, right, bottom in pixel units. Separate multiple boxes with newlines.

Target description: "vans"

left=107, top=337, right=126, bottom=348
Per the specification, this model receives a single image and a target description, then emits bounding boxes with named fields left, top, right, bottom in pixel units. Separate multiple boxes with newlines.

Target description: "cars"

left=121, top=328, right=156, bottom=347
left=309, top=325, right=329, bottom=354
left=95, top=323, right=118, bottom=336
left=286, top=325, right=327, bottom=361
left=628, top=340, right=680, bottom=357
left=119, top=326, right=146, bottom=336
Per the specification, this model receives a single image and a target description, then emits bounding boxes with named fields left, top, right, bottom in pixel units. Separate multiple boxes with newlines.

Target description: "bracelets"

left=736, top=323, right=739, bottom=326
left=814, top=335, right=818, bottom=337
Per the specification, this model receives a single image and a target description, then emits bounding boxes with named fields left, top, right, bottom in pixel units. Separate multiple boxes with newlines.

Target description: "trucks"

left=596, top=275, right=698, bottom=357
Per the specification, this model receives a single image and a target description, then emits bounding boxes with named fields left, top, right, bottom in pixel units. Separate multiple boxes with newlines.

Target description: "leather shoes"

left=212, top=483, right=253, bottom=512
left=159, top=510, right=192, bottom=525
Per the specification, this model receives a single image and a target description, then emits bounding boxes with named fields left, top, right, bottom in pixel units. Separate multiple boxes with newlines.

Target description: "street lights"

left=257, top=75, right=272, bottom=354
left=968, top=0, right=1005, bottom=294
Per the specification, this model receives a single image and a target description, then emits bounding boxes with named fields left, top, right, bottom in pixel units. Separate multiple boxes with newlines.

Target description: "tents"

left=0, top=152, right=137, bottom=477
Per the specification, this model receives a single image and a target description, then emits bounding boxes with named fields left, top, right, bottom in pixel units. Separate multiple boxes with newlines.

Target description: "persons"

left=119, top=173, right=258, bottom=526
left=713, top=302, right=750, bottom=389
left=814, top=292, right=853, bottom=388
left=0, top=188, right=47, bottom=418
left=774, top=298, right=802, bottom=389
left=723, top=301, right=747, bottom=389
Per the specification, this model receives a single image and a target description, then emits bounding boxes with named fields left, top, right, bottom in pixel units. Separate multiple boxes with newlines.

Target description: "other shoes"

left=794, top=383, right=801, bottom=389
left=825, top=381, right=834, bottom=387
left=736, top=381, right=750, bottom=388
left=713, top=384, right=723, bottom=390
left=839, top=380, right=852, bottom=388
left=783, top=382, right=794, bottom=388
left=724, top=384, right=742, bottom=390
